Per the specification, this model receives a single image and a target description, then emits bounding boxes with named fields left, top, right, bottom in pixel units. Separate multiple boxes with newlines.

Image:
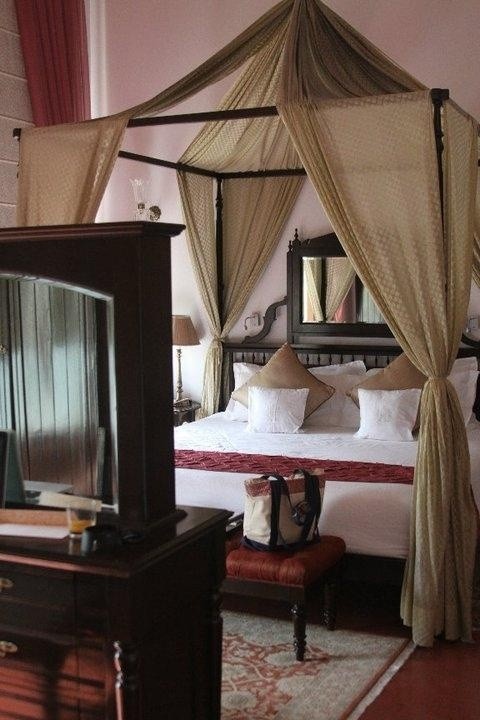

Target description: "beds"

left=12, top=0, right=480, bottom=648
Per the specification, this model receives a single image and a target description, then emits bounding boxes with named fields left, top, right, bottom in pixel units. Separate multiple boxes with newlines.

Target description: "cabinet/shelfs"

left=0, top=219, right=235, bottom=720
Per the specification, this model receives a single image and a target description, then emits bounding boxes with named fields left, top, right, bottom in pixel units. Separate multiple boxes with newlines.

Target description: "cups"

left=65, top=498, right=98, bottom=541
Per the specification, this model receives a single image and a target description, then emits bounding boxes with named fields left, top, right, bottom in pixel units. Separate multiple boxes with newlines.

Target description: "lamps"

left=129, top=176, right=161, bottom=220
left=171, top=314, right=201, bottom=405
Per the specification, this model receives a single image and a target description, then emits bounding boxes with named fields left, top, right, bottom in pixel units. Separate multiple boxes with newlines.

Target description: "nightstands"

left=173, top=398, right=201, bottom=427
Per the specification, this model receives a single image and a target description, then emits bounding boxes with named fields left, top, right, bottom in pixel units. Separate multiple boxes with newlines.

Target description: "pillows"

left=447, top=370, right=480, bottom=426
left=354, top=387, right=422, bottom=440
left=224, top=359, right=367, bottom=425
left=346, top=352, right=428, bottom=432
left=248, top=386, right=310, bottom=434
left=231, top=341, right=335, bottom=421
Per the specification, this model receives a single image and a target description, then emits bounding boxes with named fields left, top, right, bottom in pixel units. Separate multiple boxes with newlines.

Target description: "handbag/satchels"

left=240, top=468, right=327, bottom=554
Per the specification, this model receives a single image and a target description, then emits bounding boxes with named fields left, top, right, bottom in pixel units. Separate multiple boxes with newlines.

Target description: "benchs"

left=219, top=527, right=346, bottom=662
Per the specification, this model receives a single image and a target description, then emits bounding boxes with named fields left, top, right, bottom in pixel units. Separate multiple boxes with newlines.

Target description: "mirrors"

left=0, top=272, right=122, bottom=517
left=292, top=228, right=397, bottom=334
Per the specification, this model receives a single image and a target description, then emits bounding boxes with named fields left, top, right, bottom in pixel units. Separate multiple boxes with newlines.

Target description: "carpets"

left=221, top=609, right=417, bottom=720
